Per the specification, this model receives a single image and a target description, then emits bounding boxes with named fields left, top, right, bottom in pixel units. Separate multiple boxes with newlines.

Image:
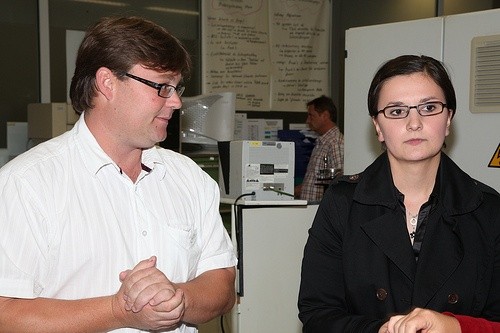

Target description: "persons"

left=298, top=54, right=500, bottom=333
left=295, top=95, right=344, bottom=204
left=394, top=308, right=500, bottom=333
left=1, top=14, right=238, bottom=333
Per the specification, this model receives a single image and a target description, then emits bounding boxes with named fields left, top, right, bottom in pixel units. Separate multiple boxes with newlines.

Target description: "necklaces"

left=397, top=201, right=432, bottom=243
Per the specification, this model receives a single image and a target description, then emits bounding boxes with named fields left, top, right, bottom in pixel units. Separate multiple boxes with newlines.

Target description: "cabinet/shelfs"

left=220, top=197, right=319, bottom=333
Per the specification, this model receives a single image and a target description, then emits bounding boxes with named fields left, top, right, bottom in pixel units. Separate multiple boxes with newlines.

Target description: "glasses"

left=110, top=69, right=184, bottom=99
left=375, top=101, right=452, bottom=118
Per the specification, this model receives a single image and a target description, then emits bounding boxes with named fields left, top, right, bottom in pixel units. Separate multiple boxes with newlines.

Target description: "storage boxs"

left=66, top=104, right=80, bottom=131
left=27, top=103, right=67, bottom=139
